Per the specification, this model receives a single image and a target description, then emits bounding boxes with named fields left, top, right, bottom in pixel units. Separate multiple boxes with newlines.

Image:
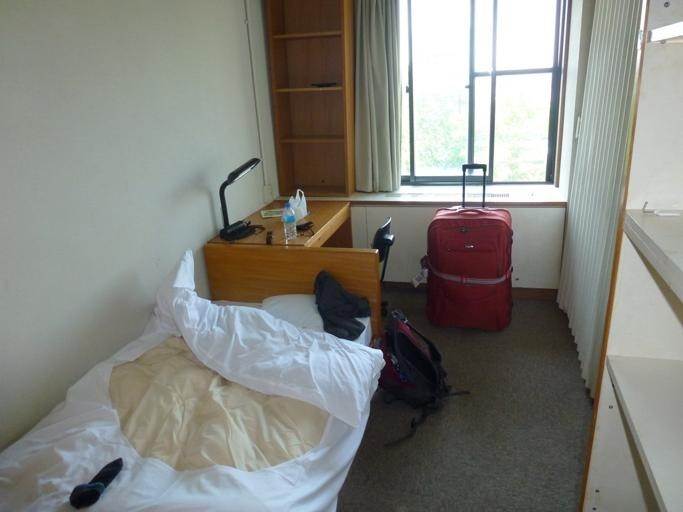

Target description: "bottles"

left=281, top=202, right=297, bottom=241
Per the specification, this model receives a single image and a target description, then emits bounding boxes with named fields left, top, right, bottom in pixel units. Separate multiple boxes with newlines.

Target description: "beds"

left=0, top=293, right=374, bottom=512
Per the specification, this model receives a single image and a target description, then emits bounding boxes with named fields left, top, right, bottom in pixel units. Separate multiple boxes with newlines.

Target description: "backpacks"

left=370, top=309, right=448, bottom=409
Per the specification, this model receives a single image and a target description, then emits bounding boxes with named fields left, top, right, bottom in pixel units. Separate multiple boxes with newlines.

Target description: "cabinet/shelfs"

left=580, top=0, right=683, bottom=511
left=204, top=199, right=381, bottom=341
left=263, top=0, right=357, bottom=199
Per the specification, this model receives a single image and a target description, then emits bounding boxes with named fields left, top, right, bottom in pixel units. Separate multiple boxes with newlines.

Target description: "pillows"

left=260, top=293, right=324, bottom=332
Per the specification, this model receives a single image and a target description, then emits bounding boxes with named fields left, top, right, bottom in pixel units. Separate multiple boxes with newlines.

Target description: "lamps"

left=217, top=151, right=263, bottom=243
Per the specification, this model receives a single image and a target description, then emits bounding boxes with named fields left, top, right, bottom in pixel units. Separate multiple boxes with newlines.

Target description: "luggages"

left=421, top=164, right=513, bottom=330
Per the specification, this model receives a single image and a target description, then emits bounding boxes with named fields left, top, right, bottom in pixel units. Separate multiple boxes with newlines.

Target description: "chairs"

left=365, top=215, right=396, bottom=297
